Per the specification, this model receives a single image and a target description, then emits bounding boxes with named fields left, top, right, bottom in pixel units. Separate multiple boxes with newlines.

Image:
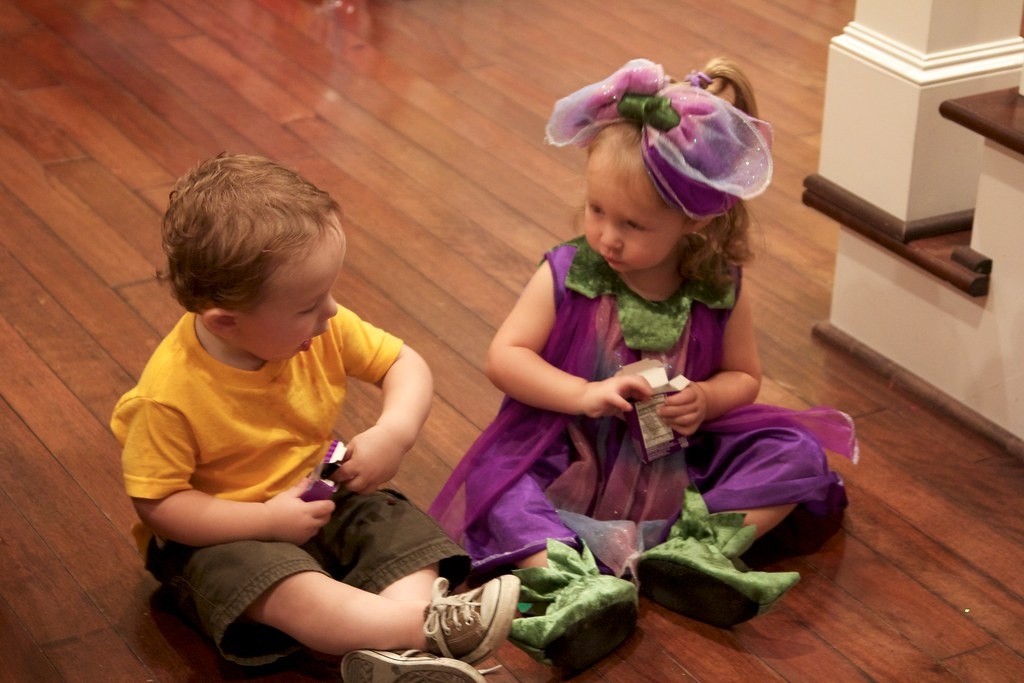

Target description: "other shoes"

left=632, top=481, right=803, bottom=629
left=509, top=539, right=639, bottom=673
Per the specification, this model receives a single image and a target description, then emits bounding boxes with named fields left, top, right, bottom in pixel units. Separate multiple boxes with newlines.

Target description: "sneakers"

left=341, top=573, right=520, bottom=683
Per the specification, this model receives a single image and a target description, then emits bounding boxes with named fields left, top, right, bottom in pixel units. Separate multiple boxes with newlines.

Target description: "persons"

left=110, top=153, right=520, bottom=683
left=424, top=54, right=848, bottom=671
